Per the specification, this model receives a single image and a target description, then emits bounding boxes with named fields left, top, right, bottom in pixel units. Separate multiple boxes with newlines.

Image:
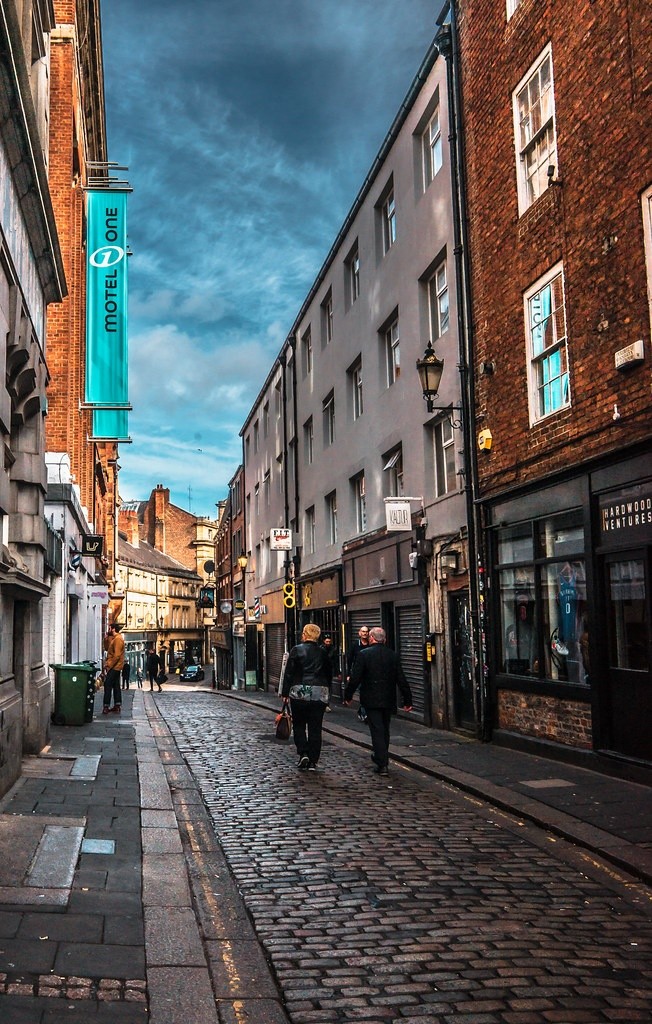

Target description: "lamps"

left=128, top=613, right=132, bottom=622
left=416, top=340, right=464, bottom=429
left=138, top=613, right=156, bottom=625
left=237, top=548, right=254, bottom=575
left=160, top=614, right=164, bottom=627
left=438, top=550, right=461, bottom=574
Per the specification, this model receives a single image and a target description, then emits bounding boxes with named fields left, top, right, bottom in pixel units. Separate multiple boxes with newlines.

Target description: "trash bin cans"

left=47, top=661, right=93, bottom=727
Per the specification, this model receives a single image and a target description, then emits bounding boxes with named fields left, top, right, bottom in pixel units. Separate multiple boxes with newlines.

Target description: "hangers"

left=516, top=566, right=535, bottom=583
left=555, top=561, right=581, bottom=580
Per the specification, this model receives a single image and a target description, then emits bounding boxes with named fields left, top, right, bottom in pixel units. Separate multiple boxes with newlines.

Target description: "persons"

left=102, top=623, right=125, bottom=713
left=148, top=648, right=162, bottom=693
left=282, top=624, right=332, bottom=771
left=137, top=667, right=143, bottom=688
left=322, top=632, right=342, bottom=713
left=347, top=626, right=369, bottom=724
left=344, top=626, right=413, bottom=775
left=122, top=660, right=129, bottom=691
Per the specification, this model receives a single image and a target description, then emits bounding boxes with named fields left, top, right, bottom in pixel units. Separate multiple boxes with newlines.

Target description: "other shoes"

left=148, top=689, right=153, bottom=692
left=102, top=706, right=122, bottom=714
left=121, top=688, right=129, bottom=690
left=357, top=714, right=368, bottom=722
left=325, top=706, right=331, bottom=712
left=157, top=688, right=163, bottom=693
left=297, top=753, right=316, bottom=770
left=371, top=753, right=389, bottom=776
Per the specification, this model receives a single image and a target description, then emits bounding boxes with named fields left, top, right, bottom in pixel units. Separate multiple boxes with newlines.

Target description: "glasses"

left=323, top=635, right=331, bottom=638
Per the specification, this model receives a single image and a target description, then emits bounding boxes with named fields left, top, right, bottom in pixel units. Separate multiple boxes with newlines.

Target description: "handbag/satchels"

left=157, top=672, right=168, bottom=684
left=275, top=701, right=293, bottom=741
